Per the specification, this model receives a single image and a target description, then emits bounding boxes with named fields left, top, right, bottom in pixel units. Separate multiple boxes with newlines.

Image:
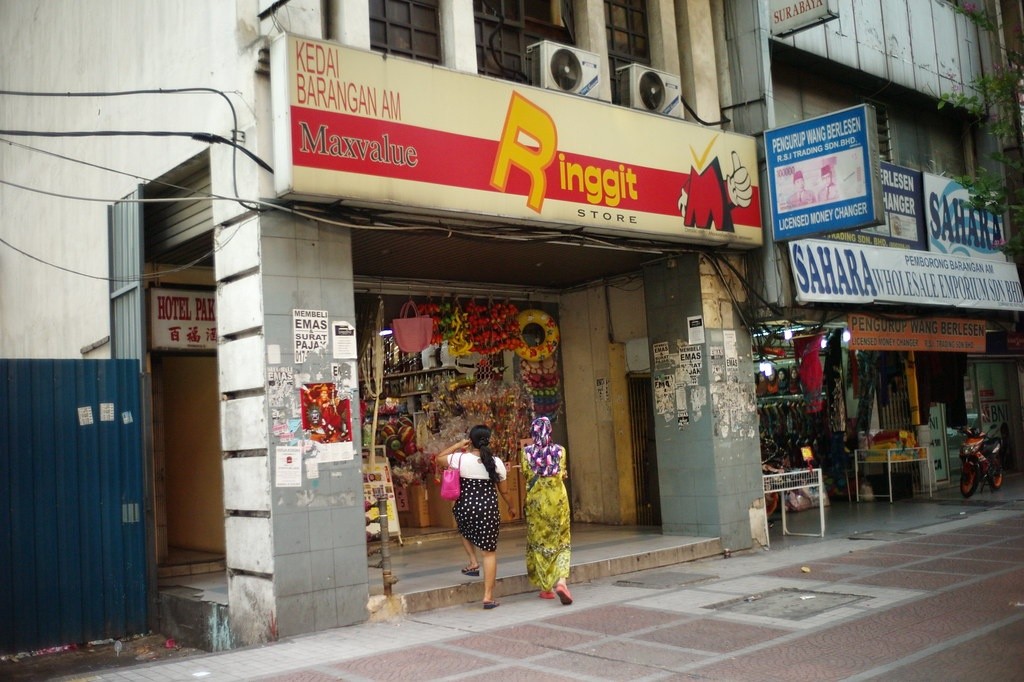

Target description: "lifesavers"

left=513, top=309, right=560, bottom=361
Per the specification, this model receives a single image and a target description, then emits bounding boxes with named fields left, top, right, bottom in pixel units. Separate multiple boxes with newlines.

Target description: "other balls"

left=381, top=415, right=417, bottom=454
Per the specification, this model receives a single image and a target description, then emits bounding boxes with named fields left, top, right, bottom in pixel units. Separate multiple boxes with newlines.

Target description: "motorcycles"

left=951, top=423, right=1005, bottom=498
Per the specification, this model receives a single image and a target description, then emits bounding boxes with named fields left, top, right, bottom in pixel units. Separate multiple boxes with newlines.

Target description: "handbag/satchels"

left=390, top=301, right=433, bottom=353
left=440, top=453, right=462, bottom=500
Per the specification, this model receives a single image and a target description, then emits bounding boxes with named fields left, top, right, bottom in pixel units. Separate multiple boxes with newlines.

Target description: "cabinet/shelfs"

left=358, top=366, right=457, bottom=417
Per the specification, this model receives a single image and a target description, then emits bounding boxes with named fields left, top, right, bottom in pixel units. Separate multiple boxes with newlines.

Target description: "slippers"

left=555, top=585, right=573, bottom=604
left=484, top=601, right=498, bottom=609
left=540, top=591, right=554, bottom=599
left=462, top=567, right=480, bottom=576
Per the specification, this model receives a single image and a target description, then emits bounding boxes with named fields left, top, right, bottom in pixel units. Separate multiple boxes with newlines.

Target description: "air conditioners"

left=615, top=62, right=685, bottom=121
left=527, top=39, right=602, bottom=99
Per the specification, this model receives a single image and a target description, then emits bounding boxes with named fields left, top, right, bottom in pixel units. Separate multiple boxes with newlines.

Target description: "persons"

left=435, top=425, right=516, bottom=609
left=1000, top=423, right=1013, bottom=469
left=754, top=367, right=799, bottom=397
left=520, top=417, right=573, bottom=605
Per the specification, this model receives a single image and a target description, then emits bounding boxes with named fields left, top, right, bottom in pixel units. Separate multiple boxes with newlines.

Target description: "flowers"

left=392, top=375, right=536, bottom=489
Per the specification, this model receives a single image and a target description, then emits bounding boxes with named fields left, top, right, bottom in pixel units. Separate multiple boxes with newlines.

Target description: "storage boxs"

left=425, top=474, right=458, bottom=527
left=394, top=484, right=431, bottom=529
left=866, top=472, right=913, bottom=502
left=494, top=437, right=533, bottom=523
